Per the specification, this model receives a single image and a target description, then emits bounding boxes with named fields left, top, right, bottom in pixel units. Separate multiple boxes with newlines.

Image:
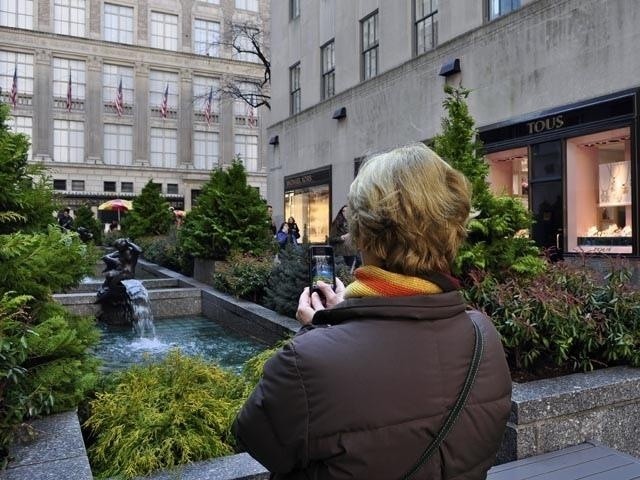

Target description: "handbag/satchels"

left=295, top=231, right=300, bottom=239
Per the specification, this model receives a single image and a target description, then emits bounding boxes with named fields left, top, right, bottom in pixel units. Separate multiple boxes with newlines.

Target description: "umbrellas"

left=173, top=209, right=187, bottom=216
left=97, top=198, right=134, bottom=224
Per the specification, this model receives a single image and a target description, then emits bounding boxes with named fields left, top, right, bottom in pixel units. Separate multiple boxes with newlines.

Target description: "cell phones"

left=308, top=245, right=336, bottom=299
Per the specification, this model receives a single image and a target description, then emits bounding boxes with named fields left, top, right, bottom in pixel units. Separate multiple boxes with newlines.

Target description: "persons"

left=230, top=144, right=513, bottom=480
left=59, top=211, right=74, bottom=233
left=110, top=220, right=118, bottom=231
left=176, top=215, right=182, bottom=228
left=266, top=205, right=300, bottom=251
left=325, top=204, right=362, bottom=269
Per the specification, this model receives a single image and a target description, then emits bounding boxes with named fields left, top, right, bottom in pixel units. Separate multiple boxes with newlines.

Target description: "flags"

left=67, top=73, right=72, bottom=113
left=114, top=80, right=123, bottom=118
left=204, top=92, right=213, bottom=127
left=248, top=98, right=253, bottom=126
left=10, top=68, right=17, bottom=109
left=161, top=86, right=168, bottom=118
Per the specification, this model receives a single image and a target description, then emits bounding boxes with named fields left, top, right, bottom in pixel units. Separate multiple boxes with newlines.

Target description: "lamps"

left=269, top=136, right=278, bottom=145
left=438, top=59, right=461, bottom=77
left=332, top=107, right=347, bottom=119
